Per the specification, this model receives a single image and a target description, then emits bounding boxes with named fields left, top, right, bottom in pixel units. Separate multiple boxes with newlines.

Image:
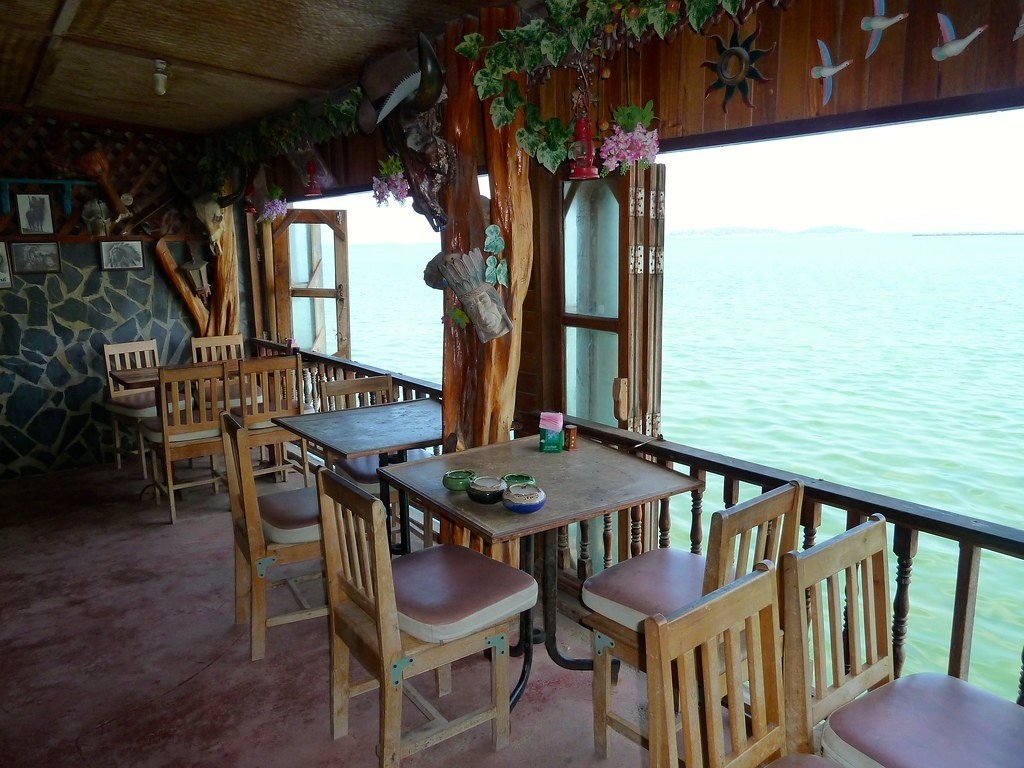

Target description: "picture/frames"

left=9, top=239, right=64, bottom=274
left=14, top=190, right=57, bottom=237
left=0, top=240, right=15, bottom=290
left=99, top=239, right=147, bottom=272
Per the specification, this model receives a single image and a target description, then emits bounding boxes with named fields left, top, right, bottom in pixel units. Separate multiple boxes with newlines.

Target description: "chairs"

left=102, top=337, right=195, bottom=479
left=219, top=411, right=371, bottom=662
left=584, top=478, right=805, bottom=768
left=650, top=559, right=846, bottom=768
left=315, top=464, right=539, bottom=768
left=231, top=352, right=311, bottom=489
left=190, top=332, right=266, bottom=463
left=138, top=361, right=231, bottom=526
left=318, top=373, right=435, bottom=559
left=784, top=512, right=1024, bottom=768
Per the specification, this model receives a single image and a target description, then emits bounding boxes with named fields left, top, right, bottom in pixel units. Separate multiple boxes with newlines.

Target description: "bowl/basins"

left=502, top=484, right=546, bottom=514
left=501, top=473, right=537, bottom=487
left=443, top=470, right=477, bottom=491
left=467, top=476, right=507, bottom=505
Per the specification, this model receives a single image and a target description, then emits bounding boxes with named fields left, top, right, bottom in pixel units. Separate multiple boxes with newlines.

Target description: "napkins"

left=539, top=412, right=564, bottom=433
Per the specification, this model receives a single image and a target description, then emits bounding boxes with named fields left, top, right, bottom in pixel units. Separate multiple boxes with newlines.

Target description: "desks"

left=378, top=431, right=706, bottom=736
left=271, top=396, right=444, bottom=560
left=109, top=355, right=318, bottom=501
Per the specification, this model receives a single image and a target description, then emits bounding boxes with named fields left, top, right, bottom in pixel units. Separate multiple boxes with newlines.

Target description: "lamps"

left=567, top=118, right=600, bottom=180
left=302, top=161, right=323, bottom=198
left=152, top=59, right=169, bottom=95
left=244, top=187, right=257, bottom=214
left=180, top=259, right=213, bottom=298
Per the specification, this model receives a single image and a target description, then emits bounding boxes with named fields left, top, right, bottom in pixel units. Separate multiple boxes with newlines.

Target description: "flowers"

left=372, top=152, right=410, bottom=203
left=253, top=184, right=287, bottom=225
left=598, top=100, right=661, bottom=179
